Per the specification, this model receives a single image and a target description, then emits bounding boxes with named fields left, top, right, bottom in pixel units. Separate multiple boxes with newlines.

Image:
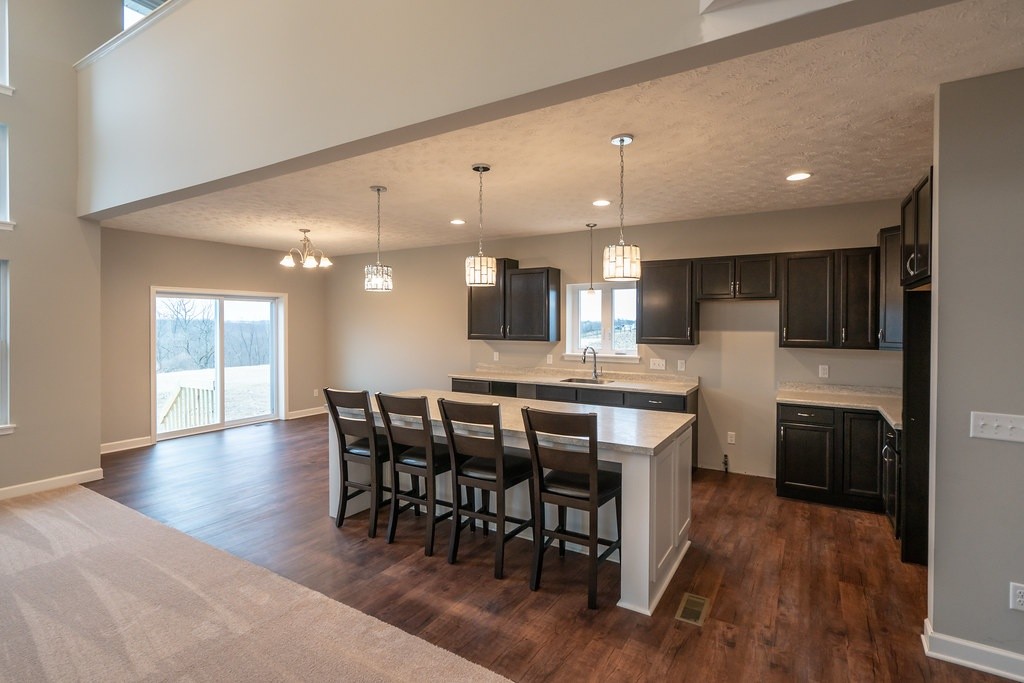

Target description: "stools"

left=325, top=388, right=622, bottom=609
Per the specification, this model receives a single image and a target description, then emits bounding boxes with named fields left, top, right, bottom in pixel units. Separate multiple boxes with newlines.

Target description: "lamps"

left=464, top=163, right=496, bottom=286
left=581, top=223, right=602, bottom=322
left=603, top=133, right=641, bottom=281
left=364, top=186, right=393, bottom=291
left=280, top=229, right=333, bottom=268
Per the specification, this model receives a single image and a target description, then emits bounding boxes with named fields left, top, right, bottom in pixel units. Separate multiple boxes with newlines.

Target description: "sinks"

left=560, top=377, right=615, bottom=384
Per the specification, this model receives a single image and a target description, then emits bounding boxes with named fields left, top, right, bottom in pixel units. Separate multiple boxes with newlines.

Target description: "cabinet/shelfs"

left=452, top=378, right=698, bottom=470
left=636, top=166, right=933, bottom=350
left=467, top=258, right=560, bottom=341
left=776, top=403, right=902, bottom=540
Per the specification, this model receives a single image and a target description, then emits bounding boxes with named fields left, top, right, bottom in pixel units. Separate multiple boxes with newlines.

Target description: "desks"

left=323, top=388, right=696, bottom=616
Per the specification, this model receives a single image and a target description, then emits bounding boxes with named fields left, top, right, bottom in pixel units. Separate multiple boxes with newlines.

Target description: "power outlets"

left=678, top=360, right=685, bottom=371
left=650, top=358, right=666, bottom=370
left=727, top=432, right=735, bottom=444
left=819, top=364, right=828, bottom=378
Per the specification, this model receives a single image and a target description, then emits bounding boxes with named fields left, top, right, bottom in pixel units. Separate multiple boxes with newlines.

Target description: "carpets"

left=0, top=485, right=517, bottom=683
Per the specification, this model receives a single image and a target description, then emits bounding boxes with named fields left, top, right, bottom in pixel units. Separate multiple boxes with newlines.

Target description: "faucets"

left=581, top=346, right=603, bottom=379
